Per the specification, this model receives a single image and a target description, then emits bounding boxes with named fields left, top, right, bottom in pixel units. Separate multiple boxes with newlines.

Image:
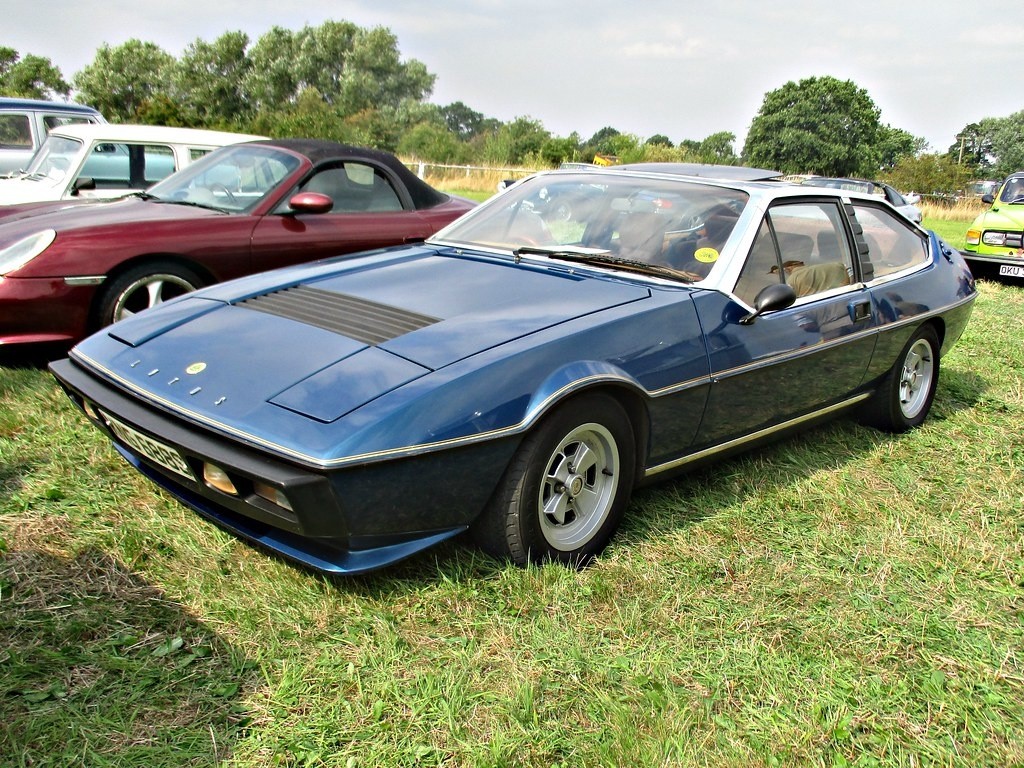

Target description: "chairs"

left=301, top=171, right=403, bottom=214
left=592, top=213, right=885, bottom=311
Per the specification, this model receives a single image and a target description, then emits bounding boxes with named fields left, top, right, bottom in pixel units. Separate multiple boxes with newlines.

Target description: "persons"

left=886, top=290, right=930, bottom=319
left=875, top=298, right=898, bottom=325
left=769, top=258, right=867, bottom=347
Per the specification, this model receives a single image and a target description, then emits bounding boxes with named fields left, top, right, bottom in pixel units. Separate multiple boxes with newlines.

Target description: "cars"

left=0, top=125, right=298, bottom=211
left=765, top=176, right=923, bottom=231
left=0, top=140, right=551, bottom=353
left=960, top=172, right=1024, bottom=287
left=47, top=163, right=978, bottom=578
left=1, top=95, right=118, bottom=172
left=493, top=164, right=706, bottom=230
